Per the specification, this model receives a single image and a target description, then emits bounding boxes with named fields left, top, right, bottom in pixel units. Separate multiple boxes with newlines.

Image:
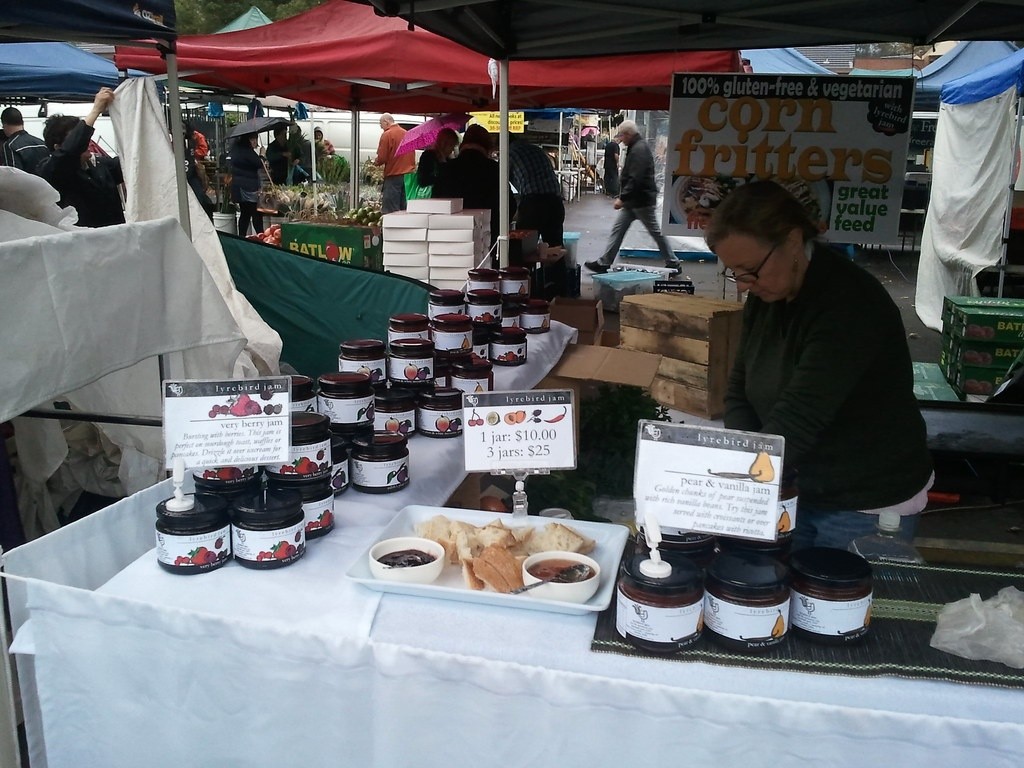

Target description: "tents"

left=915, top=48, right=1024, bottom=333
left=113, top=0, right=837, bottom=210
left=911, top=40, right=1020, bottom=105
left=0, top=0, right=192, bottom=242
left=0, top=43, right=153, bottom=104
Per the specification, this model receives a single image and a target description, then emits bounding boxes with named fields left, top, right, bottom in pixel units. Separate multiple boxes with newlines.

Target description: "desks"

left=93, top=319, right=1024, bottom=725
left=281, top=219, right=383, bottom=272
left=553, top=170, right=578, bottom=204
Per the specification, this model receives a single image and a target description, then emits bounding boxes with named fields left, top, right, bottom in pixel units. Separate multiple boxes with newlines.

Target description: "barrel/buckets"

left=213, top=211, right=236, bottom=235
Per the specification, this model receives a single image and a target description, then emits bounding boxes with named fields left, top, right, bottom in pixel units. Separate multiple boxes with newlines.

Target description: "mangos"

left=343, top=207, right=382, bottom=226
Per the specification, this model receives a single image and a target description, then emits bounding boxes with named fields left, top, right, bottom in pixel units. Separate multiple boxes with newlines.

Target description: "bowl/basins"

left=523, top=550, right=600, bottom=604
left=368, top=537, right=444, bottom=583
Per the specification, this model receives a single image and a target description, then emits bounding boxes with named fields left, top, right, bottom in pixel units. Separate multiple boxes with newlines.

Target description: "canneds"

left=616, top=467, right=874, bottom=652
left=156, top=268, right=551, bottom=575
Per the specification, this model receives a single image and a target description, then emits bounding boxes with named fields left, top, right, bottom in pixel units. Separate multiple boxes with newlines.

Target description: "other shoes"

left=664, top=264, right=683, bottom=278
left=585, top=260, right=611, bottom=273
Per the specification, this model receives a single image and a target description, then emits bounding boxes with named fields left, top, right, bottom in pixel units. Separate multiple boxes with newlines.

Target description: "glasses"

left=720, top=242, right=780, bottom=283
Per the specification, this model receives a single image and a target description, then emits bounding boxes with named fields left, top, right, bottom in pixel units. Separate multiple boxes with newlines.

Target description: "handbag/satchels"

left=239, top=188, right=261, bottom=203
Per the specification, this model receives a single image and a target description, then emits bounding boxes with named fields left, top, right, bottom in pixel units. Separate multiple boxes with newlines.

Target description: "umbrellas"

left=223, top=116, right=293, bottom=139
left=393, top=112, right=474, bottom=159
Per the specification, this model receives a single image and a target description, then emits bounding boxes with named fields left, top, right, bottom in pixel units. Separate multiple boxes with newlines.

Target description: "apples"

left=246, top=225, right=282, bottom=247
left=964, top=325, right=993, bottom=394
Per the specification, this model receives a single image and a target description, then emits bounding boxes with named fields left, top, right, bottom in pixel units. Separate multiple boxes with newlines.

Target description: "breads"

left=413, top=516, right=594, bottom=592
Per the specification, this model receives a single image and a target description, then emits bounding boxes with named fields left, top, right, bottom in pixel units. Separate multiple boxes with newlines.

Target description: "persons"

left=295, top=125, right=312, bottom=185
left=431, top=124, right=517, bottom=257
left=702, top=179, right=934, bottom=551
left=33, top=87, right=126, bottom=228
left=584, top=120, right=683, bottom=278
left=232, top=132, right=266, bottom=237
left=418, top=128, right=458, bottom=188
left=265, top=126, right=301, bottom=185
left=375, top=113, right=415, bottom=213
left=603, top=134, right=623, bottom=198
left=496, top=130, right=565, bottom=248
left=314, top=126, right=335, bottom=154
left=0, top=107, right=50, bottom=173
left=190, top=125, right=208, bottom=159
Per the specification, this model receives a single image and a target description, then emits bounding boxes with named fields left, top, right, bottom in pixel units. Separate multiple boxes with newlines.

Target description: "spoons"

left=511, top=564, right=590, bottom=595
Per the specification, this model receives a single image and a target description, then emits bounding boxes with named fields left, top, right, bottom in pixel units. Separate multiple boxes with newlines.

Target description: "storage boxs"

left=381, top=199, right=1023, bottom=421
left=906, top=172, right=931, bottom=187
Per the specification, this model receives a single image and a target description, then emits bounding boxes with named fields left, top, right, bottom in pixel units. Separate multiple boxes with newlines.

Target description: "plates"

left=671, top=174, right=829, bottom=226
